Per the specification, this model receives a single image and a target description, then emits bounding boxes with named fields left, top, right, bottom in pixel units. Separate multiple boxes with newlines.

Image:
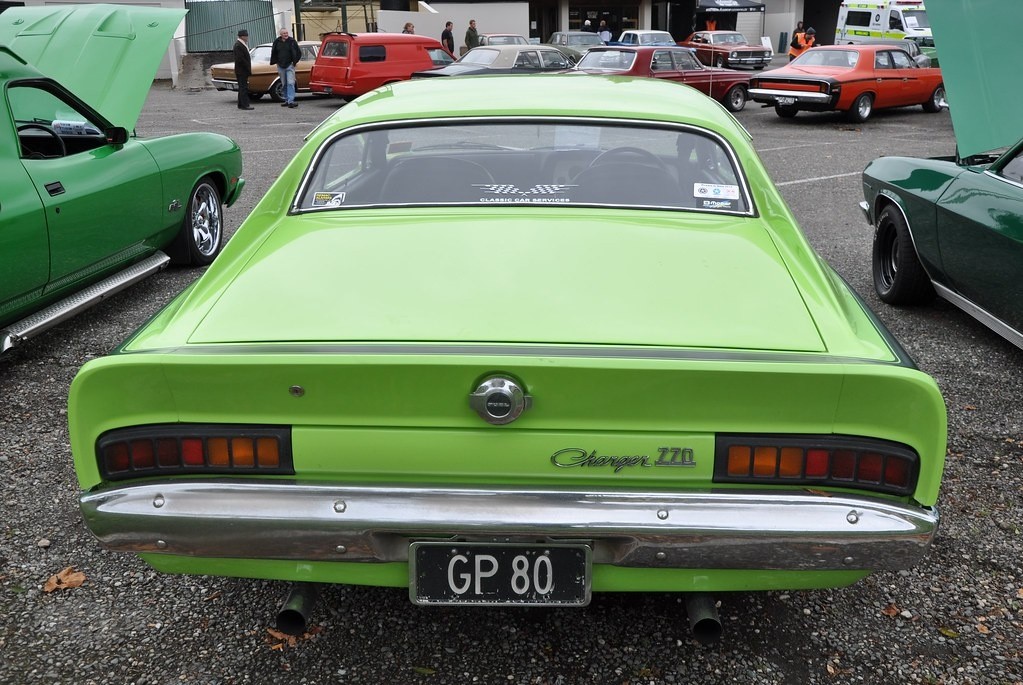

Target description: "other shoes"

left=281, top=101, right=288, bottom=107
left=242, top=105, right=254, bottom=110
left=288, top=102, right=298, bottom=108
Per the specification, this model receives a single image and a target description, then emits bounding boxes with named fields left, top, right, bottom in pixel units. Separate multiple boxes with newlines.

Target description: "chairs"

left=378, top=156, right=498, bottom=202
left=565, top=161, right=690, bottom=209
left=827, top=58, right=845, bottom=66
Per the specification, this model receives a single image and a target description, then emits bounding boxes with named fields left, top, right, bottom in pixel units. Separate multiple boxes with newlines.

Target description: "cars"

left=861, top=1, right=1023, bottom=353
left=66, top=71, right=951, bottom=616
left=2, top=3, right=248, bottom=368
left=206, top=2, right=945, bottom=125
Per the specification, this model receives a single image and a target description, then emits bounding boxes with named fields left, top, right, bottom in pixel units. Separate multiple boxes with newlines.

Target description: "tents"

left=692, top=0, right=765, bottom=36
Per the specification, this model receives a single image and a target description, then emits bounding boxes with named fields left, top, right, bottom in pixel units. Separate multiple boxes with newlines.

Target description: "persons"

left=581, top=20, right=592, bottom=32
left=233, top=29, right=254, bottom=110
left=704, top=14, right=719, bottom=30
left=402, top=23, right=414, bottom=34
left=610, top=31, right=620, bottom=41
left=465, top=20, right=480, bottom=50
left=269, top=28, right=301, bottom=108
left=792, top=21, right=805, bottom=40
left=597, top=20, right=612, bottom=43
left=789, top=27, right=817, bottom=62
left=442, top=22, right=454, bottom=54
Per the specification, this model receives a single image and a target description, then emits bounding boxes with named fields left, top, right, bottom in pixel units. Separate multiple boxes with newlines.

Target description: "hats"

left=807, top=27, right=816, bottom=35
left=237, top=30, right=248, bottom=36
